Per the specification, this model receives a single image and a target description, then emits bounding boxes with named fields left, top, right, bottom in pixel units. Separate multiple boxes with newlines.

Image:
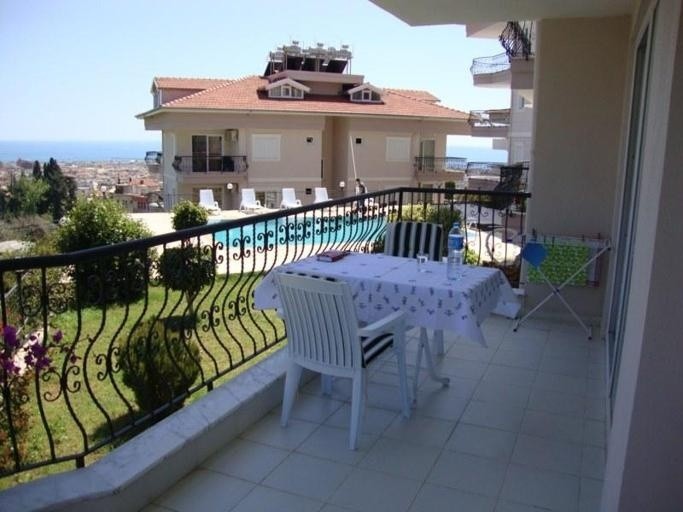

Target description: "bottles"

left=447, top=222, right=464, bottom=280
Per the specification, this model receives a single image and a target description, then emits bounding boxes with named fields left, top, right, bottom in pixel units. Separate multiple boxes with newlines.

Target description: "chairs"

left=196, top=187, right=333, bottom=215
left=274, top=272, right=412, bottom=452
left=383, top=222, right=445, bottom=265
left=468, top=161, right=524, bottom=231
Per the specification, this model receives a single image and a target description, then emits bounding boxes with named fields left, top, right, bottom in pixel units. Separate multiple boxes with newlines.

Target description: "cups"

left=416, top=254, right=428, bottom=271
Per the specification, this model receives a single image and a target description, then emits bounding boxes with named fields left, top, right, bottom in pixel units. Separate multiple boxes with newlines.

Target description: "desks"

left=276, top=251, right=502, bottom=394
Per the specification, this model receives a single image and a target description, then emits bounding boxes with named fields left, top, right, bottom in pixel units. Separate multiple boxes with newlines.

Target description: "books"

left=317, top=249, right=347, bottom=261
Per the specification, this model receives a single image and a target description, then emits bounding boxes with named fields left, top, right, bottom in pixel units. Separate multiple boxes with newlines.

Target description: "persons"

left=352, top=177, right=367, bottom=214
